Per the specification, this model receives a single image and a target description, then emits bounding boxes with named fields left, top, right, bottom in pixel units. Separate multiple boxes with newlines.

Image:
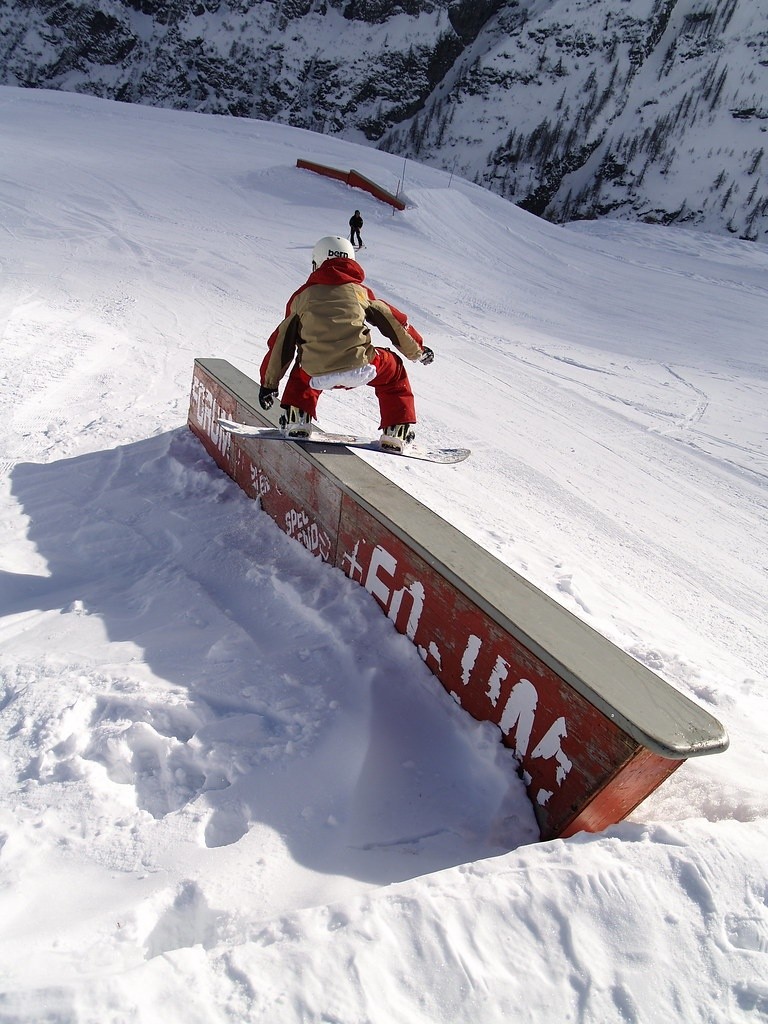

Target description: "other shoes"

left=278, top=403, right=314, bottom=439
left=380, top=418, right=415, bottom=455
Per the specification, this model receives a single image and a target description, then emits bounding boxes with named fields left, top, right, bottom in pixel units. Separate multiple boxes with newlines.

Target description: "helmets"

left=311, top=235, right=355, bottom=272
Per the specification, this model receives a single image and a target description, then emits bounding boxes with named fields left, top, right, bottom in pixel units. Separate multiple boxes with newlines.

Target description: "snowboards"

left=211, top=411, right=477, bottom=472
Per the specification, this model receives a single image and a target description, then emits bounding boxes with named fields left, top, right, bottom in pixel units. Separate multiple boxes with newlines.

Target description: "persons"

left=259, top=236, right=434, bottom=453
left=349, top=210, right=363, bottom=248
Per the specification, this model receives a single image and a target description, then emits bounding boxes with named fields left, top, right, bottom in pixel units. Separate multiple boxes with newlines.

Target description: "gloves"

left=417, top=346, right=435, bottom=366
left=259, top=385, right=279, bottom=411
